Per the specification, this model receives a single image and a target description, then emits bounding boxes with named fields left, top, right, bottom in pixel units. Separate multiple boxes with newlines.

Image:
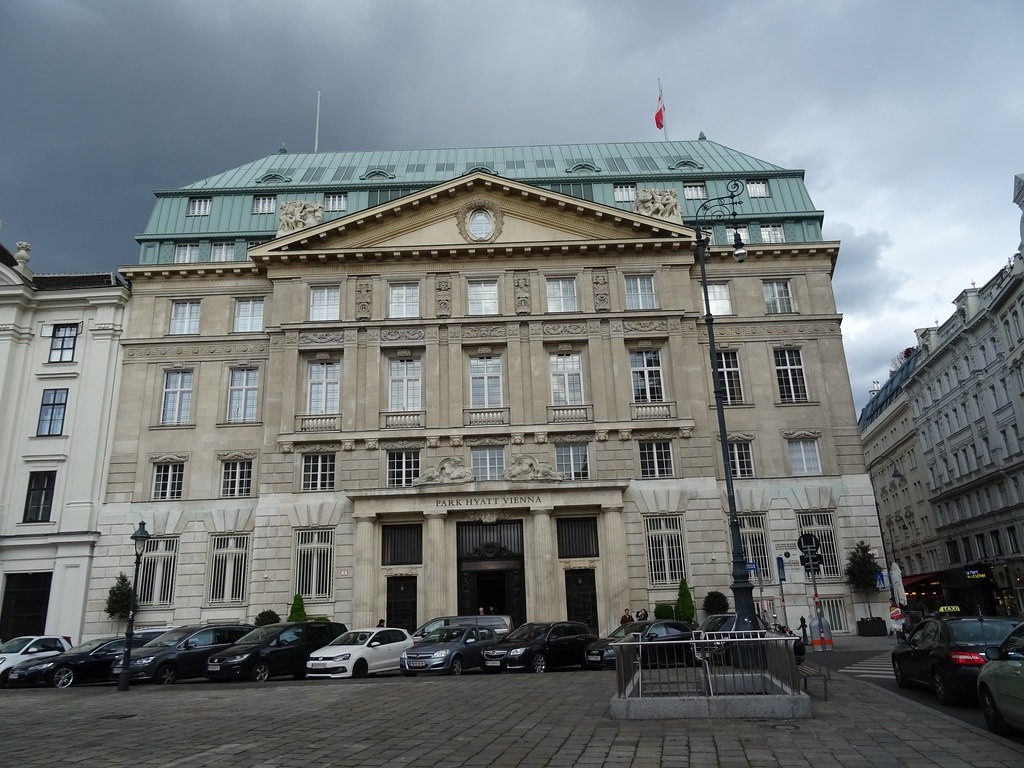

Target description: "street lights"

left=695, top=179, right=768, bottom=670
left=117, top=520, right=152, bottom=690
left=946, top=528, right=982, bottom=618
left=885, top=516, right=908, bottom=565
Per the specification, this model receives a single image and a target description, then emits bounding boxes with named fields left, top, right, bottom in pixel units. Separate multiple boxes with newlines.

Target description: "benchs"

left=797, top=662, right=828, bottom=701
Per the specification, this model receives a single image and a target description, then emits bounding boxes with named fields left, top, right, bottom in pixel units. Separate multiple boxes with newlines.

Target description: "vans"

left=409, top=616, right=515, bottom=644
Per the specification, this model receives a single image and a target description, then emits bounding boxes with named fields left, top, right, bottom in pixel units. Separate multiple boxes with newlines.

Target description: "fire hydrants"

left=800, top=616, right=811, bottom=646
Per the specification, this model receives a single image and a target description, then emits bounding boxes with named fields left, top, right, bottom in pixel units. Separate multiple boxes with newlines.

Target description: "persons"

left=477, top=608, right=487, bottom=616
left=620, top=608, right=648, bottom=634
left=376, top=619, right=386, bottom=627
left=887, top=597, right=898, bottom=639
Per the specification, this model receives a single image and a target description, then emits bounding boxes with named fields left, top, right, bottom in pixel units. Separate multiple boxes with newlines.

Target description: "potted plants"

left=840, top=540, right=888, bottom=637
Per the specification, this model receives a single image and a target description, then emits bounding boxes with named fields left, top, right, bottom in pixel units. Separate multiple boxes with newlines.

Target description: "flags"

left=654, top=80, right=666, bottom=129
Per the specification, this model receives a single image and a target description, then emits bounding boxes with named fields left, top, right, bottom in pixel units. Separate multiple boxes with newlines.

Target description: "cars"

left=109, top=623, right=261, bottom=684
left=203, top=623, right=350, bottom=681
left=691, top=614, right=806, bottom=664
left=480, top=622, right=600, bottom=673
left=583, top=619, right=696, bottom=669
left=399, top=625, right=500, bottom=675
left=128, top=626, right=215, bottom=645
left=975, top=622, right=1024, bottom=736
left=7, top=636, right=151, bottom=688
left=891, top=605, right=1024, bottom=705
left=303, top=628, right=415, bottom=678
left=0, top=636, right=72, bottom=686
